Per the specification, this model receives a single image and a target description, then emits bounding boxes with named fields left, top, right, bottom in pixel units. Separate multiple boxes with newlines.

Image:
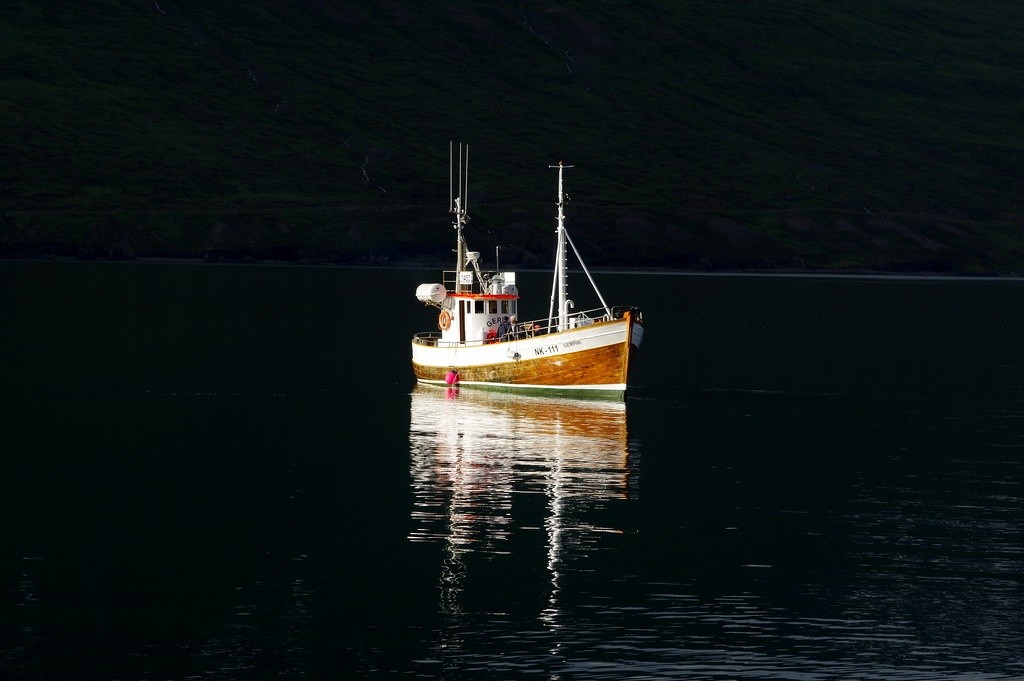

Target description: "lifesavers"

left=439, top=311, right=451, bottom=330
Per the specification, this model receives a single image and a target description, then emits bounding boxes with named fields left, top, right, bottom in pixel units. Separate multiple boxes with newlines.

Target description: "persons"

left=497, top=316, right=512, bottom=342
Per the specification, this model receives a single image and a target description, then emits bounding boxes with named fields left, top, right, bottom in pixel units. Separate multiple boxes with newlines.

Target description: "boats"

left=407, top=383, right=629, bottom=651
left=411, top=142, right=633, bottom=394
